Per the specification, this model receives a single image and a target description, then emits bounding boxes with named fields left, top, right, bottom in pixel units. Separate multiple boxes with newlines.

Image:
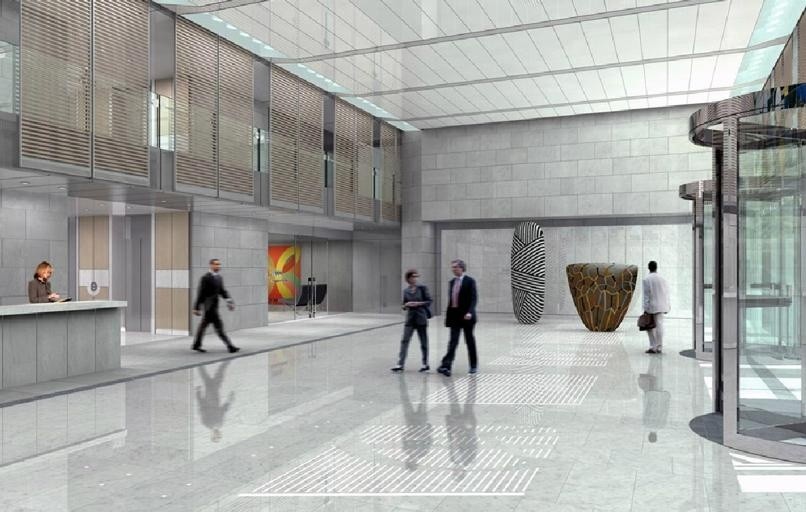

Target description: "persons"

left=28, top=261, right=59, bottom=302
left=642, top=261, right=668, bottom=353
left=638, top=352, right=671, bottom=441
left=193, top=353, right=237, bottom=442
left=395, top=369, right=434, bottom=469
left=437, top=259, right=478, bottom=375
left=439, top=371, right=480, bottom=481
left=190, top=259, right=239, bottom=353
left=390, top=272, right=432, bottom=372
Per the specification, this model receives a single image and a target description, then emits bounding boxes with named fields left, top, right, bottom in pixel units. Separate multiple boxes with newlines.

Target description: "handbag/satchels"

left=426, top=306, right=432, bottom=318
left=638, top=312, right=656, bottom=331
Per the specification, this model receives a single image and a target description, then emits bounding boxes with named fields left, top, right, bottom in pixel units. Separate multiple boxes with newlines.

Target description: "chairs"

left=294, top=283, right=327, bottom=317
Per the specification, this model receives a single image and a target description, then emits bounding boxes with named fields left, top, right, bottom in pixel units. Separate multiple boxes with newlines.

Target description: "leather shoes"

left=391, top=368, right=403, bottom=370
left=645, top=349, right=656, bottom=353
left=193, top=348, right=207, bottom=352
left=657, top=350, right=661, bottom=353
left=230, top=347, right=240, bottom=353
left=419, top=366, right=429, bottom=371
left=470, top=368, right=476, bottom=373
left=438, top=367, right=450, bottom=376
